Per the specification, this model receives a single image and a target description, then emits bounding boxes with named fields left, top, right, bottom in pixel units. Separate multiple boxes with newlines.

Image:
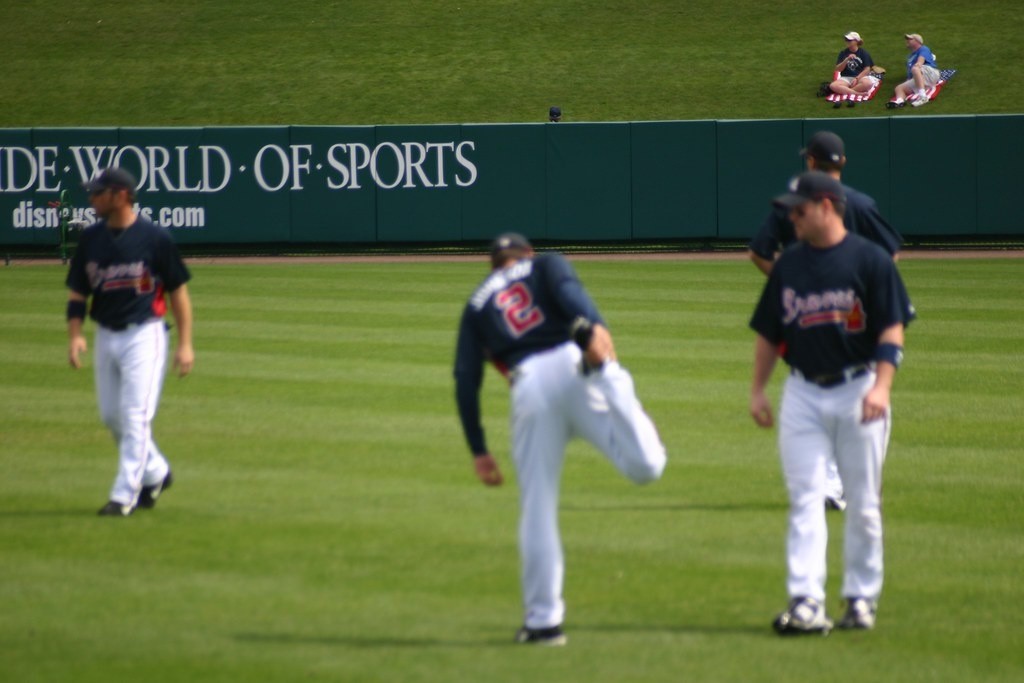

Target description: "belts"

left=105, top=317, right=147, bottom=333
left=790, top=366, right=869, bottom=388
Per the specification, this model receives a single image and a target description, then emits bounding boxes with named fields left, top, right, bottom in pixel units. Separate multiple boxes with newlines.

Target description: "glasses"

left=783, top=202, right=823, bottom=219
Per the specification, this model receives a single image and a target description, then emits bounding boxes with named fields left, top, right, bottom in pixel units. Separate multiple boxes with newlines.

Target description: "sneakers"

left=911, top=95, right=930, bottom=108
left=884, top=100, right=907, bottom=110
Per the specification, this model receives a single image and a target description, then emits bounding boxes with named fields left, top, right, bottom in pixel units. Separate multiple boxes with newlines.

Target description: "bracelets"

left=856, top=78, right=858, bottom=82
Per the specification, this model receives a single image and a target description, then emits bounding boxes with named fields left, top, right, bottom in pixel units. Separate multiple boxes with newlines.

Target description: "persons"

left=747, top=170, right=918, bottom=636
left=830, top=31, right=873, bottom=95
left=65, top=167, right=195, bottom=516
left=885, top=33, right=940, bottom=110
left=454, top=231, right=666, bottom=647
left=549, top=107, right=562, bottom=122
left=749, top=131, right=904, bottom=510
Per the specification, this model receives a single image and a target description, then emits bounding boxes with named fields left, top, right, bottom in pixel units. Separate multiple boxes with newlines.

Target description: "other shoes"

left=98, top=498, right=140, bottom=518
left=513, top=622, right=566, bottom=649
left=773, top=596, right=832, bottom=636
left=846, top=100, right=855, bottom=108
left=827, top=490, right=847, bottom=511
left=138, top=471, right=173, bottom=509
left=571, top=317, right=607, bottom=380
left=835, top=596, right=876, bottom=632
left=833, top=101, right=842, bottom=110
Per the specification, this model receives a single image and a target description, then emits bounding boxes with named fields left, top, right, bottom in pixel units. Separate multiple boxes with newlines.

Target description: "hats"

left=772, top=169, right=847, bottom=208
left=491, top=233, right=529, bottom=257
left=799, top=131, right=847, bottom=162
left=87, top=167, right=136, bottom=198
left=844, top=32, right=861, bottom=41
left=905, top=34, right=923, bottom=45
left=550, top=107, right=561, bottom=118
